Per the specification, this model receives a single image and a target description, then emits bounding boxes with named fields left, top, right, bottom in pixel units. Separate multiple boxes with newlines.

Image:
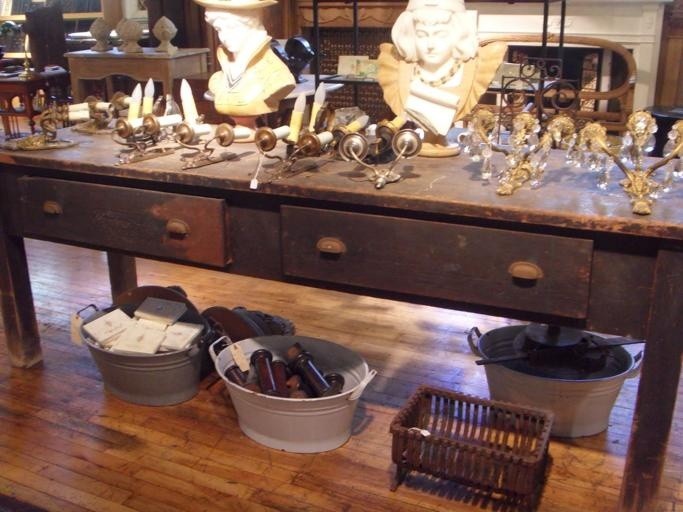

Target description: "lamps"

left=17, top=77, right=683, bottom=214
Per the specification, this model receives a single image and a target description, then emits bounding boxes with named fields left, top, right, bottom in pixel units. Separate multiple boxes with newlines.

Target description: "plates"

left=113, top=284, right=199, bottom=320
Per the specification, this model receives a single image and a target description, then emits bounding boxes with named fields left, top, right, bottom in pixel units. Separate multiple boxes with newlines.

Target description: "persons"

left=376, top=0, right=508, bottom=158
left=195, top=0, right=297, bottom=118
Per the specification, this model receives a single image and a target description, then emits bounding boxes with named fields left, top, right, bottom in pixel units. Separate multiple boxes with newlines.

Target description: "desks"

left=64, top=47, right=209, bottom=104
left=0, top=69, right=70, bottom=136
left=0, top=124, right=683, bottom=512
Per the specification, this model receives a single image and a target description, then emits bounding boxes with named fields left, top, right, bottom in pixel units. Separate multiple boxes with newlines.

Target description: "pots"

left=72, top=304, right=214, bottom=409
left=468, top=322, right=643, bottom=442
left=205, top=333, right=379, bottom=454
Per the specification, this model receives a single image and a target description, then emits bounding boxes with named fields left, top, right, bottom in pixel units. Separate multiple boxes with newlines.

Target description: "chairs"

left=0, top=18, right=31, bottom=72
left=22, top=5, right=72, bottom=100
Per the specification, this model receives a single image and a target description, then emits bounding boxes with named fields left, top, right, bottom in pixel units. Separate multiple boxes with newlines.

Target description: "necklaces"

left=412, top=54, right=463, bottom=88
